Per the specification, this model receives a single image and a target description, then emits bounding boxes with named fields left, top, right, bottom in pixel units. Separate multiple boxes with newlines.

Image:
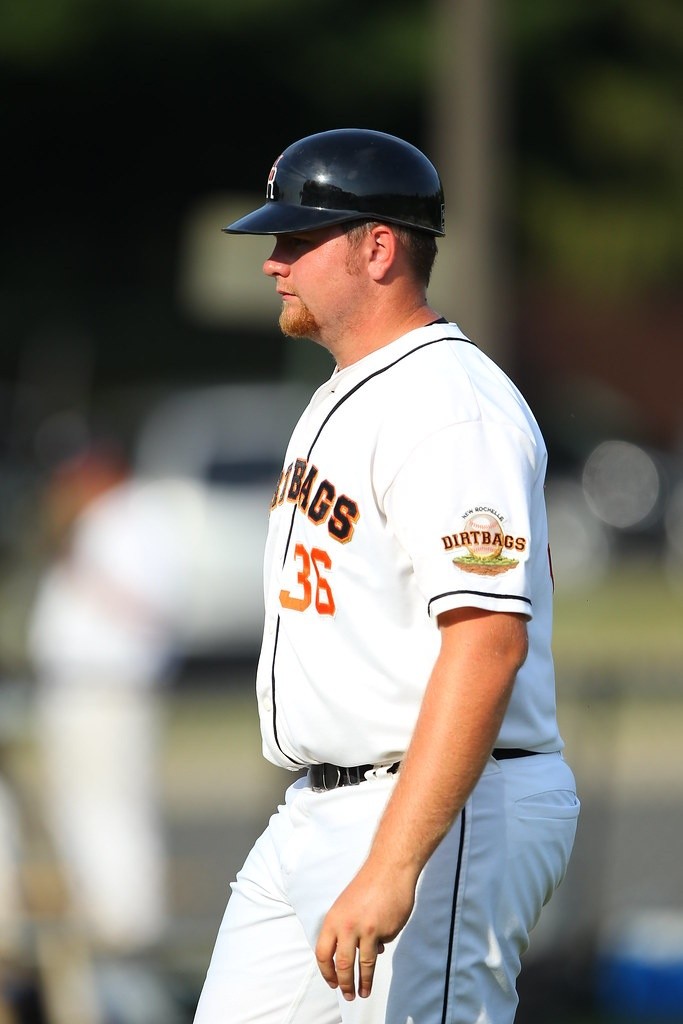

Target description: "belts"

left=308, top=746, right=546, bottom=795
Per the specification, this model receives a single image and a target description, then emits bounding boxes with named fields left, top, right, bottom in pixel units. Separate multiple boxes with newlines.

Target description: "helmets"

left=218, top=126, right=448, bottom=241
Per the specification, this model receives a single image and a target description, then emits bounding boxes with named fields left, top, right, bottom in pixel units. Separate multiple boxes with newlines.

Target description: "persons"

left=173, top=128, right=586, bottom=1024
